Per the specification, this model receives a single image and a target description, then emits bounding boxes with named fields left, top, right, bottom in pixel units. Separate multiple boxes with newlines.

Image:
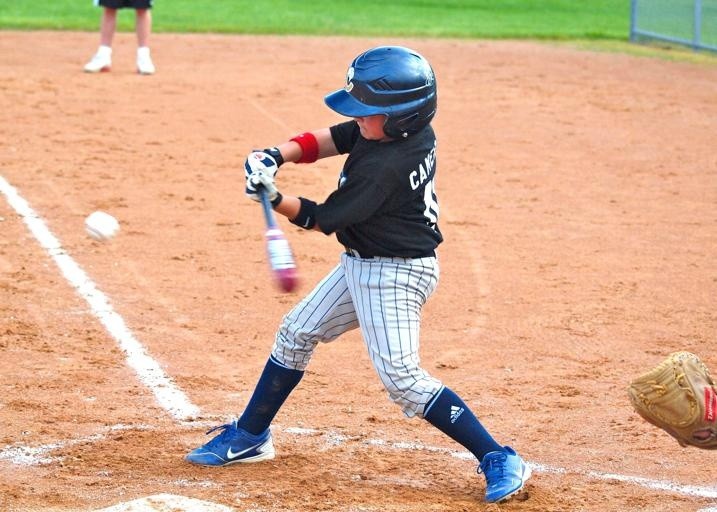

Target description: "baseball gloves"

left=628, top=351, right=717, bottom=452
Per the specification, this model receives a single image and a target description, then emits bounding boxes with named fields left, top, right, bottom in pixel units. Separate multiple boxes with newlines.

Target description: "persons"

left=184, top=45, right=533, bottom=504
left=83, top=0, right=155, bottom=75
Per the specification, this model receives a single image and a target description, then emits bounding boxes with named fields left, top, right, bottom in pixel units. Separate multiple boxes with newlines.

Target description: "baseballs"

left=84, top=210, right=120, bottom=239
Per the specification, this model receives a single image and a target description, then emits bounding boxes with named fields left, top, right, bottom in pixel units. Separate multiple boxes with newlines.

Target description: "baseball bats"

left=258, top=185, right=299, bottom=293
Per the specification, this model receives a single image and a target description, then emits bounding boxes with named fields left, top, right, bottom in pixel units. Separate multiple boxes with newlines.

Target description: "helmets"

left=321, top=42, right=440, bottom=141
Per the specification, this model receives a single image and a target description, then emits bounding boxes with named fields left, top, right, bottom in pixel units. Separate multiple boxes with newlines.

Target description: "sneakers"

left=477, top=443, right=535, bottom=504
left=182, top=420, right=279, bottom=467
left=79, top=45, right=113, bottom=74
left=135, top=47, right=157, bottom=76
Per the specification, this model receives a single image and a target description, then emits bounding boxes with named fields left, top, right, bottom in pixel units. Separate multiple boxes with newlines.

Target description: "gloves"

left=244, top=173, right=284, bottom=207
left=241, top=144, right=286, bottom=183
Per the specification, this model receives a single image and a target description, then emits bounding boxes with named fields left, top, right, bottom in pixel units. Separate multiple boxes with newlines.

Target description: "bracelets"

left=288, top=132, right=320, bottom=163
left=288, top=196, right=318, bottom=231
